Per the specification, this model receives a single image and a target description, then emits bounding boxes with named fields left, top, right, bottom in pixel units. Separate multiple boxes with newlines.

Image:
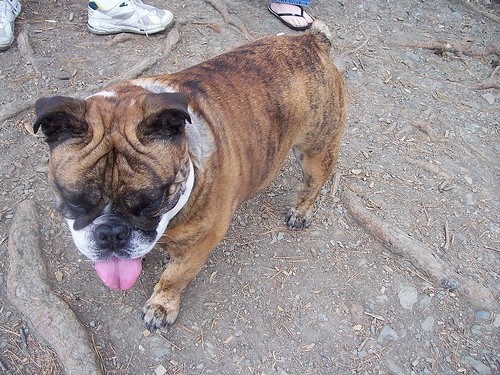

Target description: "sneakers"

left=87, top=0, right=173, bottom=40
left=0, top=0, right=21, bottom=50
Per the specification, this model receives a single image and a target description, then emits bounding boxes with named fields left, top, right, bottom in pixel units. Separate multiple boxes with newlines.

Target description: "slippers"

left=268, top=1, right=314, bottom=30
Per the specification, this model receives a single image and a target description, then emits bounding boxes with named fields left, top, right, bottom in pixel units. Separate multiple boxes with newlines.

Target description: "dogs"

left=30, top=17, right=348, bottom=335
